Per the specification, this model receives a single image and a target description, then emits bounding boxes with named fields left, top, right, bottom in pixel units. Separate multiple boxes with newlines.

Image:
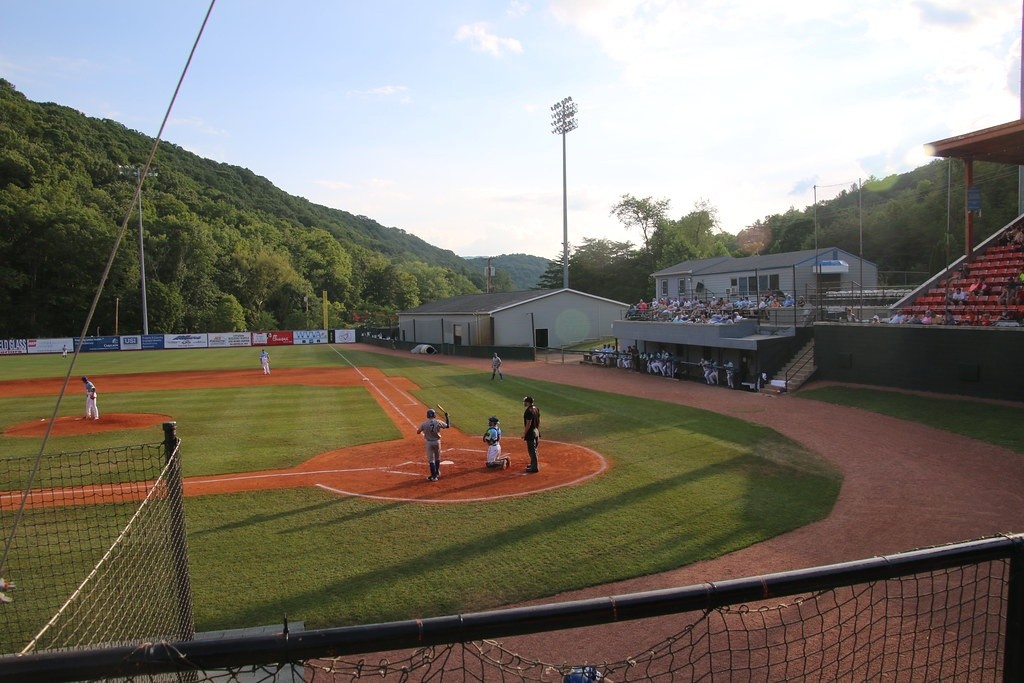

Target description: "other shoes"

left=526, top=464, right=538, bottom=472
left=500, top=457, right=511, bottom=470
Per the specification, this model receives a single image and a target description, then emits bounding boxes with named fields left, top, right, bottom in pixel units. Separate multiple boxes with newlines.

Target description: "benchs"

left=584, top=354, right=620, bottom=362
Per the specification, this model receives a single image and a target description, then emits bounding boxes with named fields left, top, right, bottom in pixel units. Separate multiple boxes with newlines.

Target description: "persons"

left=416, top=409, right=451, bottom=482
left=624, top=288, right=807, bottom=326
left=483, top=415, right=511, bottom=470
left=723, top=359, right=736, bottom=389
left=492, top=352, right=504, bottom=382
left=593, top=343, right=678, bottom=378
left=520, top=396, right=540, bottom=473
left=830, top=308, right=934, bottom=326
left=259, top=348, right=272, bottom=376
left=937, top=263, right=1024, bottom=328
left=81, top=375, right=98, bottom=421
left=700, top=358, right=719, bottom=385
left=1000, top=223, right=1024, bottom=249
left=62, top=344, right=68, bottom=359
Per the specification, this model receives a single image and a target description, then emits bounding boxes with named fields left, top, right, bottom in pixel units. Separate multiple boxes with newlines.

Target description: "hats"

left=488, top=418, right=499, bottom=423
left=522, top=397, right=534, bottom=403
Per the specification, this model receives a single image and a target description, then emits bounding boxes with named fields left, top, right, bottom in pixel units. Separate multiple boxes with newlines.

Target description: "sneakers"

left=436, top=471, right=441, bottom=477
left=426, top=476, right=438, bottom=481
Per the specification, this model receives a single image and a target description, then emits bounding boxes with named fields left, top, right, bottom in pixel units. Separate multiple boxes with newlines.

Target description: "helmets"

left=427, top=409, right=436, bottom=418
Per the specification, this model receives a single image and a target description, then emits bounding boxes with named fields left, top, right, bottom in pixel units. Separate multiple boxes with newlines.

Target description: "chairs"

left=898, top=223, right=1024, bottom=325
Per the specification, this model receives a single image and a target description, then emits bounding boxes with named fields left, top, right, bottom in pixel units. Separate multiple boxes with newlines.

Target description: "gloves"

left=445, top=413, right=448, bottom=417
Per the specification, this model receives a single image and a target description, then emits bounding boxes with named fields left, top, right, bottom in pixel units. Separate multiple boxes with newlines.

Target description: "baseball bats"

left=436, top=404, right=450, bottom=416
